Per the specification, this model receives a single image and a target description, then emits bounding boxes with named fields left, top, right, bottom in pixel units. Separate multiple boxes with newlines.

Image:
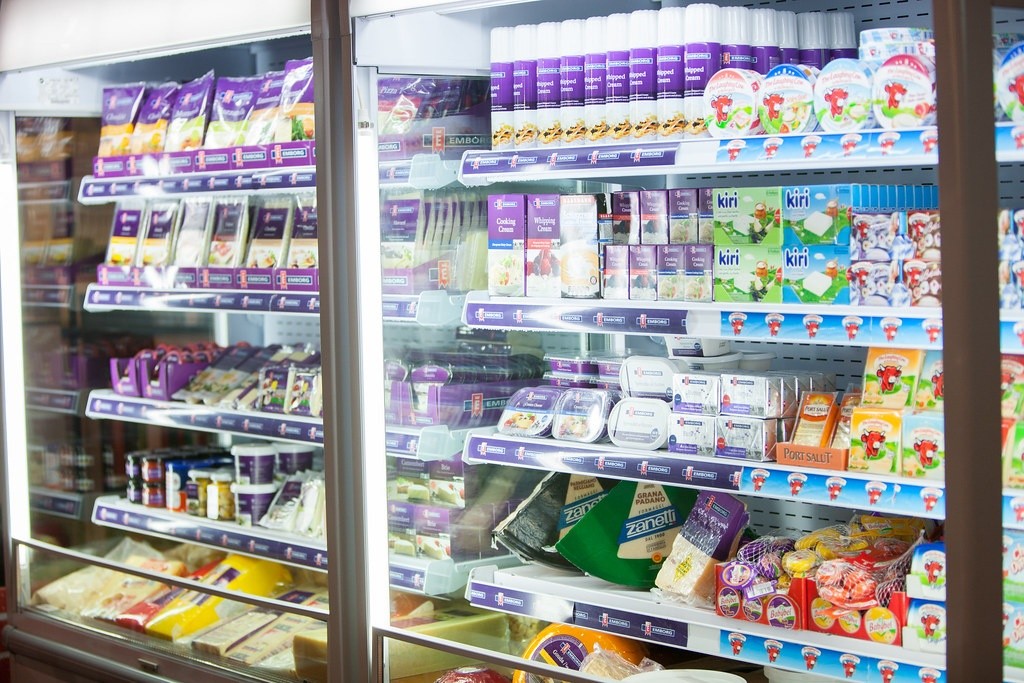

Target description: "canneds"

left=123, top=442, right=312, bottom=530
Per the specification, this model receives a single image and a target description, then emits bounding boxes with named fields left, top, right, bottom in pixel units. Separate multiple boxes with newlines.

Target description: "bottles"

left=485, top=2, right=858, bottom=151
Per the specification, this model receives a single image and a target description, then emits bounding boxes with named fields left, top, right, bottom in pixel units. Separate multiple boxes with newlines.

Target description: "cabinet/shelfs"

left=1, top=0, right=1023, bottom=683
